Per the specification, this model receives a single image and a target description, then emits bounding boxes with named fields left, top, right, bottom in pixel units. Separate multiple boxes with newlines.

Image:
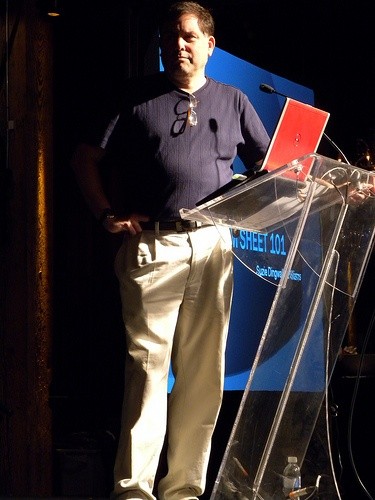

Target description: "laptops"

left=196, top=97, right=330, bottom=232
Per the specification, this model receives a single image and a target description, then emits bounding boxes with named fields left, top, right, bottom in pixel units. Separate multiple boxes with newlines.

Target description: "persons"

left=74, top=0, right=374, bottom=500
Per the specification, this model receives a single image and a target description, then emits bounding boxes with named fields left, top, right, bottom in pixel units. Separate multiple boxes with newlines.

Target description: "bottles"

left=355, top=146, right=374, bottom=192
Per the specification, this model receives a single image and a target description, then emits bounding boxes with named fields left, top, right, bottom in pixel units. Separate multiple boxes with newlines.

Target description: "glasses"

left=186, top=92, right=200, bottom=128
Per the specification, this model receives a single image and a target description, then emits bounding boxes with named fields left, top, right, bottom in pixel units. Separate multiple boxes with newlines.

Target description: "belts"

left=138, top=221, right=212, bottom=234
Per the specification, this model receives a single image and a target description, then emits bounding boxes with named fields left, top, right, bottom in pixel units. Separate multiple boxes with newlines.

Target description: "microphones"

left=260, top=83, right=350, bottom=165
